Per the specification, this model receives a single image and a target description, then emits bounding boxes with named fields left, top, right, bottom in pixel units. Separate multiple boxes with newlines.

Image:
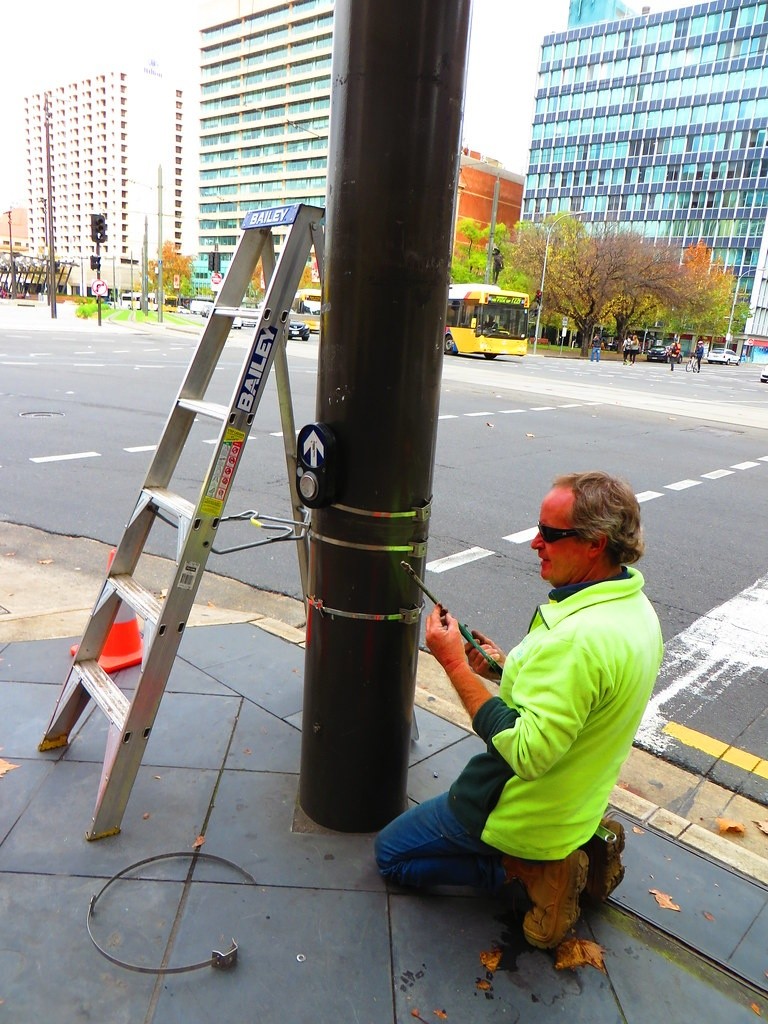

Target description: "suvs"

left=646, top=337, right=682, bottom=364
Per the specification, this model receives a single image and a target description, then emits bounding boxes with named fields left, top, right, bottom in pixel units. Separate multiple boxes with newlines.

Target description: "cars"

left=760, top=365, right=768, bottom=383
left=707, top=347, right=740, bottom=366
left=179, top=300, right=310, bottom=342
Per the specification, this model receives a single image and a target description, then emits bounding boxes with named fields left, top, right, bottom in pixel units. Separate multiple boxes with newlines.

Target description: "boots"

left=577, top=817, right=625, bottom=901
left=502, top=845, right=588, bottom=949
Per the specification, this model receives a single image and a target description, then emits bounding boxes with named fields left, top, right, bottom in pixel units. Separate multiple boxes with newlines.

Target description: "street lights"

left=532, top=211, right=587, bottom=356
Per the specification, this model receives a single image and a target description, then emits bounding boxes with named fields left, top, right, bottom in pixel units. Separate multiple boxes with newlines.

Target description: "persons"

left=492, top=248, right=503, bottom=286
left=695, top=341, right=703, bottom=373
left=373, top=472, right=663, bottom=949
left=485, top=314, right=498, bottom=329
left=670, top=338, right=681, bottom=371
left=621, top=335, right=639, bottom=365
left=591, top=334, right=601, bottom=362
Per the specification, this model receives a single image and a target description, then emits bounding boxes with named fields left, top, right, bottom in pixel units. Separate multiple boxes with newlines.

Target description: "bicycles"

left=685, top=352, right=698, bottom=373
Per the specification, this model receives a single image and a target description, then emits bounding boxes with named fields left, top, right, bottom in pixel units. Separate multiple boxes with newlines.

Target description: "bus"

left=291, top=289, right=322, bottom=333
left=444, top=284, right=530, bottom=360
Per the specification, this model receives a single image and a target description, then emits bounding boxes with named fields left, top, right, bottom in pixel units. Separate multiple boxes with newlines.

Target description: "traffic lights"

left=535, top=289, right=542, bottom=306
left=91, top=215, right=105, bottom=243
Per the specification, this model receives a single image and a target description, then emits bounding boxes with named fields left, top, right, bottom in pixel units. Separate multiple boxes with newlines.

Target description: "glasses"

left=537, top=521, right=586, bottom=544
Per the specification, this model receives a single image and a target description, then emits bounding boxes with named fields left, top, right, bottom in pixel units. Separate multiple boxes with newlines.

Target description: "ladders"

left=39, top=204, right=326, bottom=842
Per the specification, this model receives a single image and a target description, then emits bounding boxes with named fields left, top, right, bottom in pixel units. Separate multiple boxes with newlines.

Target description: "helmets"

left=674, top=338, right=678, bottom=342
left=698, top=341, right=703, bottom=343
